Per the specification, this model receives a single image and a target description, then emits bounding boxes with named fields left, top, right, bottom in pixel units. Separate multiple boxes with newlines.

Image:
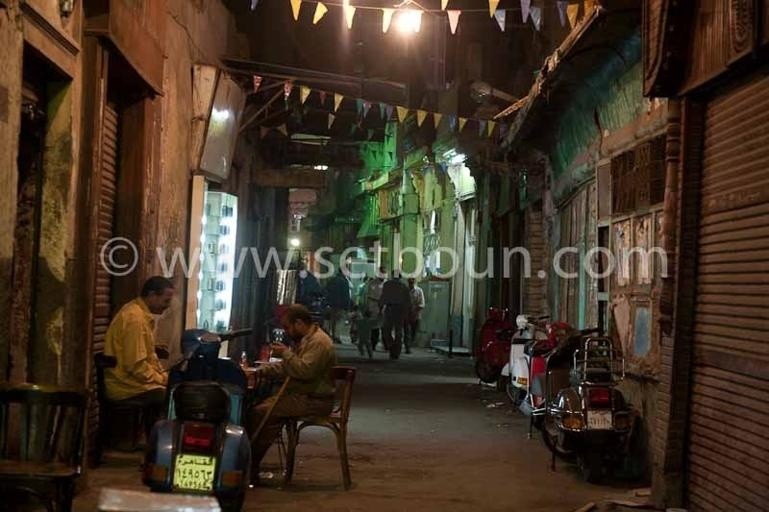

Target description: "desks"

left=239, top=363, right=282, bottom=481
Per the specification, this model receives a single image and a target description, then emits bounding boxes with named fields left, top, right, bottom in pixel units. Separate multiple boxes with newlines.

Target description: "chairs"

left=1, top=383, right=87, bottom=511
left=90, top=339, right=169, bottom=468
left=277, top=360, right=353, bottom=490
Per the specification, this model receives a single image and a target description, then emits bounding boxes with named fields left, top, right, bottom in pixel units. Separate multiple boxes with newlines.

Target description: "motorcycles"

left=138, top=327, right=256, bottom=512
left=474, top=306, right=640, bottom=482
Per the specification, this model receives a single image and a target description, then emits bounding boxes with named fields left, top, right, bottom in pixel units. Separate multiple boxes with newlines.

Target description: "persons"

left=101, top=275, right=177, bottom=445
left=321, top=265, right=356, bottom=343
left=240, top=301, right=339, bottom=480
left=351, top=265, right=427, bottom=361
